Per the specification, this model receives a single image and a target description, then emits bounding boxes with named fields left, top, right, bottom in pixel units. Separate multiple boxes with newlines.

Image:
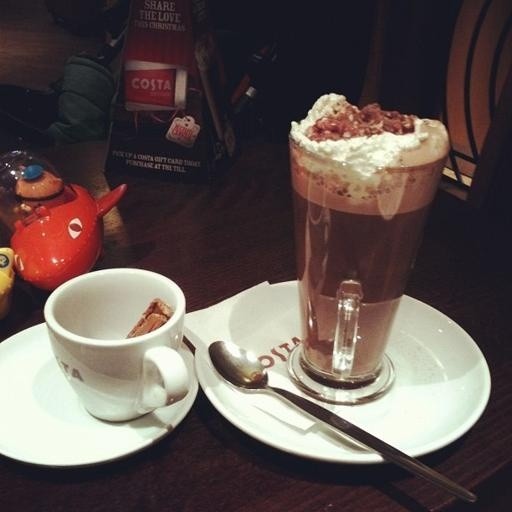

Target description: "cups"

left=287, top=119, right=451, bottom=408
left=44, top=266, right=190, bottom=424
left=1, top=150, right=70, bottom=232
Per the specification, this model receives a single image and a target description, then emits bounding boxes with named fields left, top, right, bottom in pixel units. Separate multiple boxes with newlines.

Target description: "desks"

left=1, top=0, right=82, bottom=135
left=0, top=129, right=512, bottom=512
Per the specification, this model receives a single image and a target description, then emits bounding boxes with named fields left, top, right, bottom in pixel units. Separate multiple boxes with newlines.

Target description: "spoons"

left=208, top=340, right=475, bottom=504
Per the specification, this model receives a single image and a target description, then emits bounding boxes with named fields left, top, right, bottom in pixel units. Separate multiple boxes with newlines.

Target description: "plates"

left=197, top=278, right=492, bottom=464
left=1, top=323, right=197, bottom=467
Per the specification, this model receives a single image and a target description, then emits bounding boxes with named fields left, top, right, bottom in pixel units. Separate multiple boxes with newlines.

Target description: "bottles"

left=16, top=166, right=66, bottom=210
